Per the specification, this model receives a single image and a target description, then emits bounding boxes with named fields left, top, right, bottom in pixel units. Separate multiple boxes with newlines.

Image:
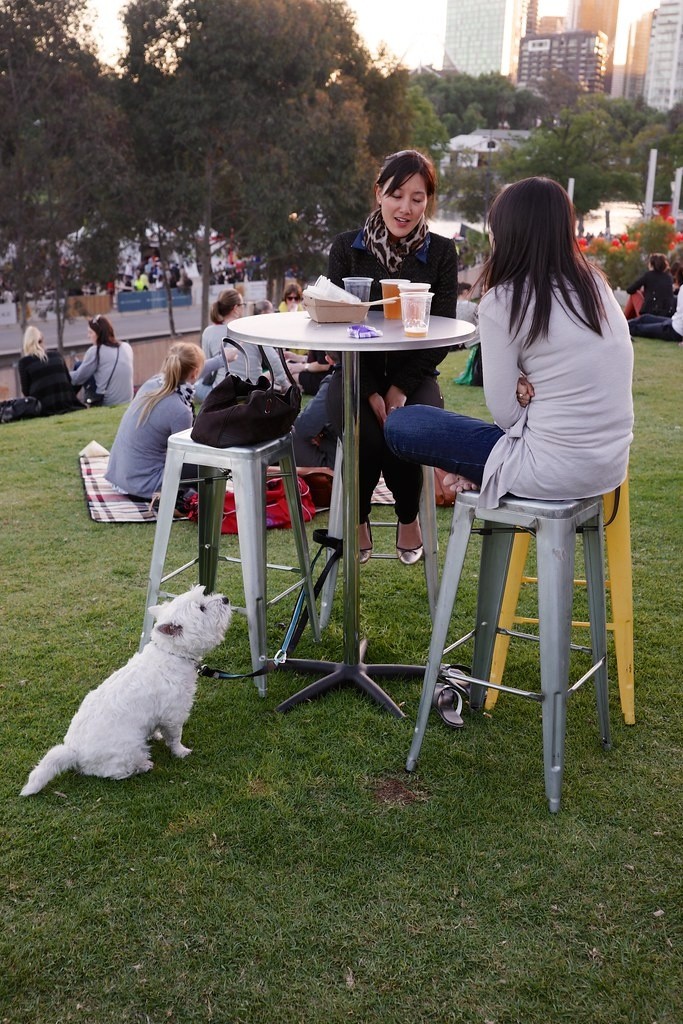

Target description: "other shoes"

left=358, top=516, right=373, bottom=564
left=395, top=514, right=424, bottom=565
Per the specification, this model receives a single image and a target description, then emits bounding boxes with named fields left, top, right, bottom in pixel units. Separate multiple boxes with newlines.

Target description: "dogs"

left=20, top=586, right=232, bottom=798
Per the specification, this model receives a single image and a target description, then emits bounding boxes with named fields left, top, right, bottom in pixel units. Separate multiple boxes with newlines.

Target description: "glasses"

left=232, top=303, right=246, bottom=309
left=93, top=314, right=101, bottom=329
left=287, top=296, right=300, bottom=301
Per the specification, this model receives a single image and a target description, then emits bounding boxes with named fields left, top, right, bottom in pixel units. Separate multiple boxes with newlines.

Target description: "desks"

left=227, top=311, right=476, bottom=714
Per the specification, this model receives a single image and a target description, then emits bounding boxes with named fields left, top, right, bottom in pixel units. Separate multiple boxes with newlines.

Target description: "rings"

left=389, top=407, right=396, bottom=410
left=519, top=393, right=523, bottom=399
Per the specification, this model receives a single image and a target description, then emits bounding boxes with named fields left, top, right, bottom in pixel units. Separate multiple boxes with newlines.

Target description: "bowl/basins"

left=302, top=298, right=370, bottom=324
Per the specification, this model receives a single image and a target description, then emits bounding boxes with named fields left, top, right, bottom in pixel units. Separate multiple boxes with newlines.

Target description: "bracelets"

left=306, top=363, right=309, bottom=372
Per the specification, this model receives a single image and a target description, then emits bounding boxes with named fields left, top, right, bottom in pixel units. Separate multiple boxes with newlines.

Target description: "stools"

left=405, top=491, right=614, bottom=811
left=486, top=472, right=636, bottom=723
left=318, top=426, right=440, bottom=632
left=136, top=426, right=318, bottom=698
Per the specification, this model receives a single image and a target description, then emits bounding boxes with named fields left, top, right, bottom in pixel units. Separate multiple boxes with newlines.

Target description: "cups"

left=399, top=291, right=435, bottom=337
left=314, top=275, right=361, bottom=303
left=379, top=279, right=411, bottom=320
left=397, top=283, right=431, bottom=293
left=342, top=276, right=374, bottom=302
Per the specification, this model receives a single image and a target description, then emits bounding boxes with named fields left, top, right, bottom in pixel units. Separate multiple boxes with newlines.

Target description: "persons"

left=623, top=252, right=683, bottom=345
left=16, top=327, right=88, bottom=416
left=456, top=282, right=490, bottom=348
left=104, top=342, right=244, bottom=500
left=186, top=283, right=331, bottom=401
left=121, top=253, right=258, bottom=292
left=68, top=315, right=134, bottom=407
left=328, top=149, right=458, bottom=566
left=386, top=177, right=633, bottom=500
left=290, top=350, right=340, bottom=470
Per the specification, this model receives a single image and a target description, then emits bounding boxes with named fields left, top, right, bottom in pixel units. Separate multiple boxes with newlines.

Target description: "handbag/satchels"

left=431, top=466, right=456, bottom=507
left=0, top=397, right=41, bottom=425
left=186, top=473, right=316, bottom=533
left=651, top=301, right=673, bottom=316
left=265, top=465, right=334, bottom=509
left=190, top=337, right=302, bottom=449
left=83, top=392, right=105, bottom=406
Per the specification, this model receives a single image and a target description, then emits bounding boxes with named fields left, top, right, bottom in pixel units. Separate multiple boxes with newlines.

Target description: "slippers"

left=437, top=661, right=489, bottom=697
left=431, top=681, right=463, bottom=726
